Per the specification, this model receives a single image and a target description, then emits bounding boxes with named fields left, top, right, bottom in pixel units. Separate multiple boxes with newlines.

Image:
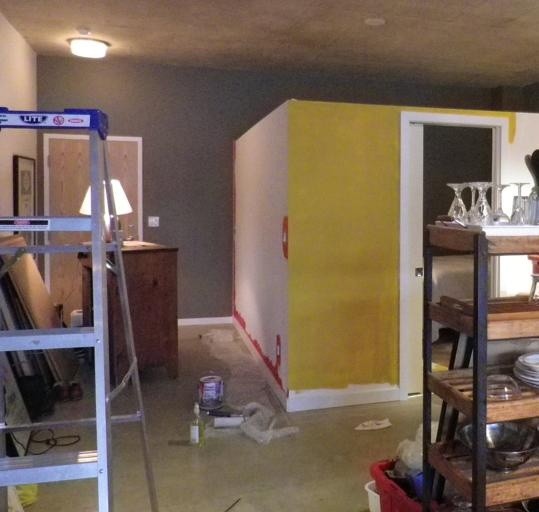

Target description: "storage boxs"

left=370, top=459, right=423, bottom=512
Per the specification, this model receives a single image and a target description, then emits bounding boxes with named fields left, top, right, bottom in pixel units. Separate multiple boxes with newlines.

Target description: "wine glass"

left=493, top=185, right=509, bottom=226
left=446, top=183, right=468, bottom=226
left=511, top=182, right=531, bottom=224
left=471, top=181, right=494, bottom=225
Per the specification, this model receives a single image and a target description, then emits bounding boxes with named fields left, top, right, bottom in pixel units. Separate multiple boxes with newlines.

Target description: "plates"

left=514, top=351, right=539, bottom=388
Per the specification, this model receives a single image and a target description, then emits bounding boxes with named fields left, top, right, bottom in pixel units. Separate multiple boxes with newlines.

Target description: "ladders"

left=0, top=106, right=162, bottom=512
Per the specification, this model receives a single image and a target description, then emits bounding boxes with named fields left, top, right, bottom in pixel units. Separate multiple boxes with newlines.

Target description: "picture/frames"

left=1, top=353, right=33, bottom=455
left=0, top=235, right=79, bottom=384
left=13, top=155, right=37, bottom=263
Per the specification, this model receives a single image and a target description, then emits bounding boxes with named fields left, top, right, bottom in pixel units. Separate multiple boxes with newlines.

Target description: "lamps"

left=69, top=39, right=110, bottom=59
left=77, top=180, right=132, bottom=243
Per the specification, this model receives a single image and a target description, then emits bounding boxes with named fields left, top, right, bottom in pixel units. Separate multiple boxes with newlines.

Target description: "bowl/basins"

left=458, top=422, right=539, bottom=475
left=484, top=375, right=520, bottom=402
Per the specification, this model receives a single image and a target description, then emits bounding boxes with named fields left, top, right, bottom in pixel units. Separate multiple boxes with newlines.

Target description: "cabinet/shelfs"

left=81, top=239, right=178, bottom=386
left=421, top=226, right=539, bottom=512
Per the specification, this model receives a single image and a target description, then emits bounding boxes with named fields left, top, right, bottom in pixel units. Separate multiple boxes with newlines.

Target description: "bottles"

left=189, top=401, right=205, bottom=448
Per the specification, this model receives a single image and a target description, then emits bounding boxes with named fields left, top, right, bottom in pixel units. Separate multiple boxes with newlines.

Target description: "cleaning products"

left=186, top=402, right=206, bottom=450
